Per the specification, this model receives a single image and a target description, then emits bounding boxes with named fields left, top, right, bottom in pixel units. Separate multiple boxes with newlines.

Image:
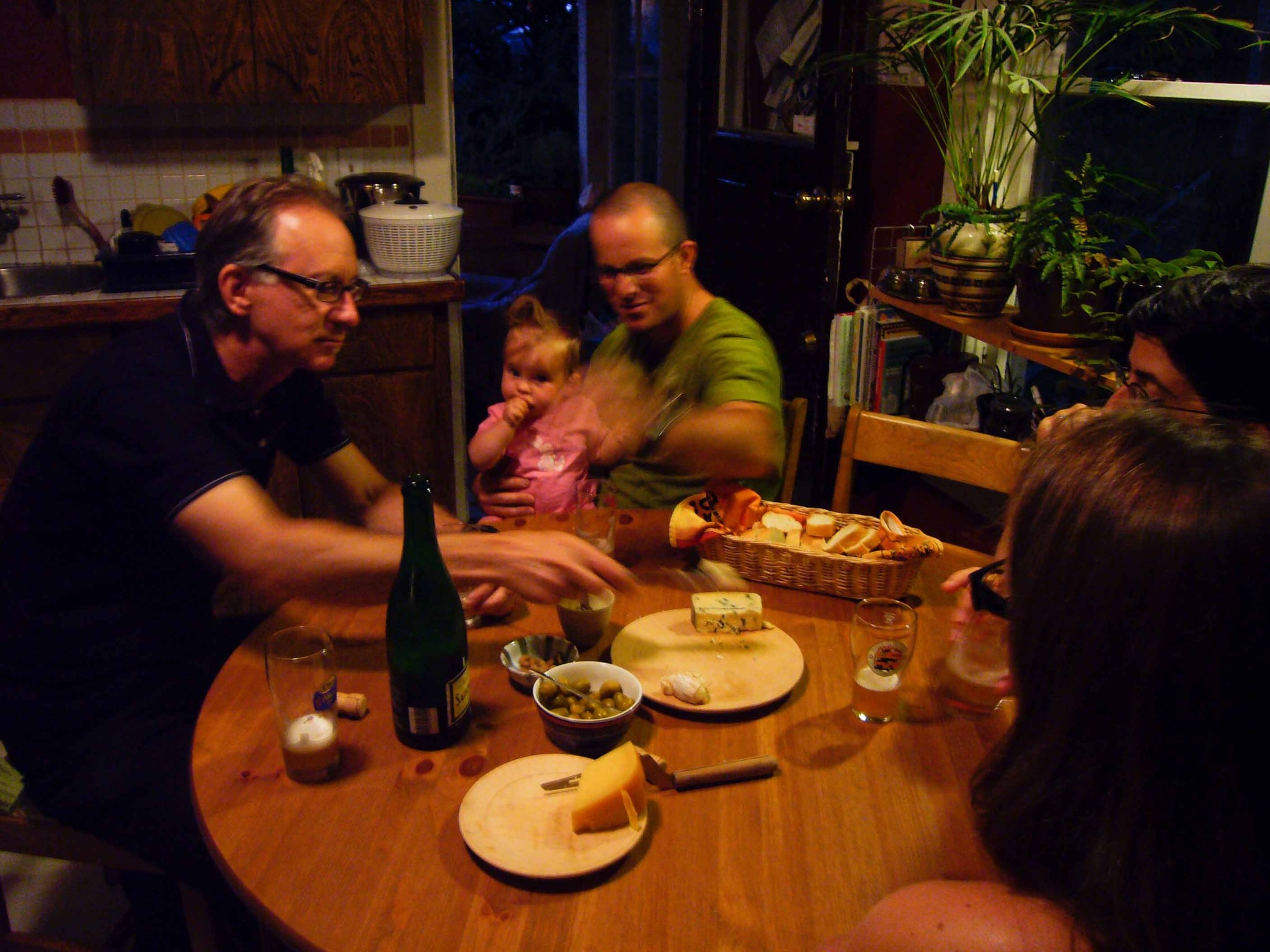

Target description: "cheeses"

left=689, top=591, right=762, bottom=633
left=662, top=670, right=709, bottom=706
left=571, top=741, right=646, bottom=835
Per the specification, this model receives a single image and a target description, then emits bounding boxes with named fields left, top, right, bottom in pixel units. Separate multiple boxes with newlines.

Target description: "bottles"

left=384, top=474, right=470, bottom=751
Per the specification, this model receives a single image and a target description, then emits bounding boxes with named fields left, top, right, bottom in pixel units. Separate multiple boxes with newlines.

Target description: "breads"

left=737, top=512, right=881, bottom=560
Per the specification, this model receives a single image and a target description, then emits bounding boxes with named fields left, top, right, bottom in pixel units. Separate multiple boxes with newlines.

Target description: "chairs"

left=774, top=396, right=1029, bottom=567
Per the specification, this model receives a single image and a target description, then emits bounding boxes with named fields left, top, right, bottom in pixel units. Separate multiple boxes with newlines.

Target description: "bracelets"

left=633, top=395, right=692, bottom=459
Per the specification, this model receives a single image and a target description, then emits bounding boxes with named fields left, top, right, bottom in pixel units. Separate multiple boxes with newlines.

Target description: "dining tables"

left=192, top=507, right=1020, bottom=952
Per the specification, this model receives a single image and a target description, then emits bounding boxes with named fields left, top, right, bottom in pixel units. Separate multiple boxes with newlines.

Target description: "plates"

left=611, top=608, right=805, bottom=714
left=459, top=754, right=648, bottom=879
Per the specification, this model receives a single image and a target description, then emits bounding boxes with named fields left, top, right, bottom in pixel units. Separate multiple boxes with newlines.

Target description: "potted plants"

left=907, top=56, right=1177, bottom=336
left=777, top=0, right=1263, bottom=317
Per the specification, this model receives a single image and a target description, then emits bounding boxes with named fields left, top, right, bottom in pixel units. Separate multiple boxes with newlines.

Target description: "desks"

left=865, top=285, right=1126, bottom=420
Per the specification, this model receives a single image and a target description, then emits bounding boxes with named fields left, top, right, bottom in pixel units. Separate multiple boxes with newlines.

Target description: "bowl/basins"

left=533, top=661, right=643, bottom=747
left=500, top=634, right=579, bottom=689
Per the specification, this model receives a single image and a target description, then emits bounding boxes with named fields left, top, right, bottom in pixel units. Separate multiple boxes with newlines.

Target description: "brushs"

left=52, top=176, right=112, bottom=256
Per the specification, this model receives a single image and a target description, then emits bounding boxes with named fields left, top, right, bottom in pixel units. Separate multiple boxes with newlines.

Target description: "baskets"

left=358, top=197, right=464, bottom=273
left=702, top=500, right=930, bottom=600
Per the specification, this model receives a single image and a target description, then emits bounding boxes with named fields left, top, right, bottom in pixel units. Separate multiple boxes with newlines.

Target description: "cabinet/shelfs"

left=682, top=0, right=950, bottom=304
left=55, top=0, right=425, bottom=139
left=0, top=300, right=456, bottom=525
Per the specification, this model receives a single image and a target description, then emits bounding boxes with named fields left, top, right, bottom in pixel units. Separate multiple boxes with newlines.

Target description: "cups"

left=934, top=574, right=1011, bottom=714
left=436, top=522, right=497, bottom=628
left=574, top=478, right=616, bottom=560
left=264, top=625, right=341, bottom=782
left=848, top=597, right=918, bottom=722
left=556, top=588, right=615, bottom=650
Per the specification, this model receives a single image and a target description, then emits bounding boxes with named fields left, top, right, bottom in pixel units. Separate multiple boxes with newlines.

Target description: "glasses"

left=596, top=239, right=685, bottom=281
left=968, top=556, right=1016, bottom=621
left=245, top=258, right=366, bottom=302
left=1115, top=365, right=1233, bottom=420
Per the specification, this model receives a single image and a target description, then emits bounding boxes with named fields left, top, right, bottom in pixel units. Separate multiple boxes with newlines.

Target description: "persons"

left=472, top=181, right=787, bottom=519
left=0, top=175, right=638, bottom=952
left=468, top=295, right=647, bottom=537
left=988, top=260, right=1270, bottom=599
left=831, top=407, right=1270, bottom=951
